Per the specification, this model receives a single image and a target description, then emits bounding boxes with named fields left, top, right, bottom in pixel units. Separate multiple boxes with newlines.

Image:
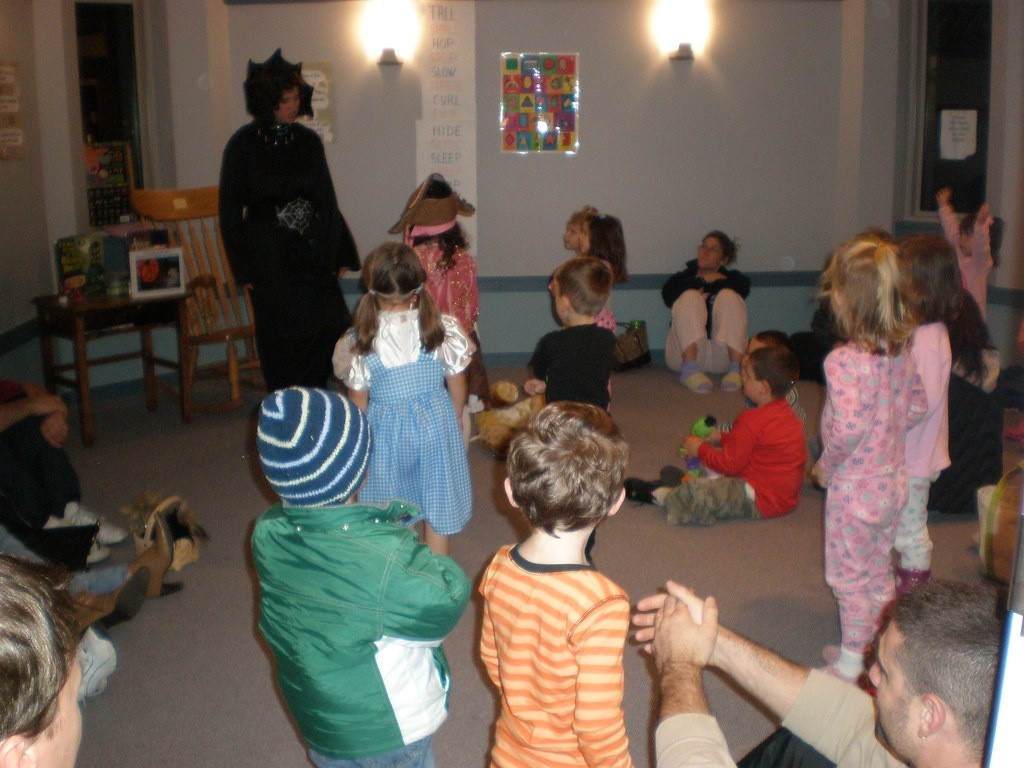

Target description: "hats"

left=388, top=172, right=476, bottom=233
left=257, top=386, right=374, bottom=509
left=245, top=48, right=314, bottom=123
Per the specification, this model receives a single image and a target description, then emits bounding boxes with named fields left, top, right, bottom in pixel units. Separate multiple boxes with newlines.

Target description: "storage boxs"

left=107, top=224, right=183, bottom=299
left=55, top=232, right=107, bottom=300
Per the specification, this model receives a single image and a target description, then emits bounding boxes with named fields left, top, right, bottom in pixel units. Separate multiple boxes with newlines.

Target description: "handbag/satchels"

left=613, top=320, right=651, bottom=373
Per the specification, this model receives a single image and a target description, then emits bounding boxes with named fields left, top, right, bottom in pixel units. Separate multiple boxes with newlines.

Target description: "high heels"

left=75, top=567, right=151, bottom=640
left=130, top=511, right=183, bottom=599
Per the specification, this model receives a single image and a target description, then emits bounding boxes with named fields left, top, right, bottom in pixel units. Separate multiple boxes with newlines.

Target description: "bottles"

left=691, top=416, right=716, bottom=437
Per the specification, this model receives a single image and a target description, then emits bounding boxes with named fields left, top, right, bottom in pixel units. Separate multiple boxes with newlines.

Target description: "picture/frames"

left=83, top=140, right=134, bottom=229
left=130, top=246, right=186, bottom=301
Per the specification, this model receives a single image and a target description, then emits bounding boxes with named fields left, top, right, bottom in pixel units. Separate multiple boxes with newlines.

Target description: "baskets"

left=131, top=497, right=202, bottom=573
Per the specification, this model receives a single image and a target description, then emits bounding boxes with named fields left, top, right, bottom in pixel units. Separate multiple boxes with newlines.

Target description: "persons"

left=249, top=386, right=471, bottom=768
left=926, top=290, right=1004, bottom=513
left=662, top=231, right=752, bottom=394
left=523, top=204, right=629, bottom=416
left=217, top=47, right=361, bottom=394
left=332, top=241, right=477, bottom=557
left=935, top=187, right=1005, bottom=321
left=0, top=550, right=82, bottom=768
left=0, top=377, right=183, bottom=704
left=632, top=579, right=1009, bottom=768
left=622, top=330, right=807, bottom=526
left=812, top=228, right=952, bottom=686
left=477, top=401, right=635, bottom=768
left=388, top=173, right=487, bottom=398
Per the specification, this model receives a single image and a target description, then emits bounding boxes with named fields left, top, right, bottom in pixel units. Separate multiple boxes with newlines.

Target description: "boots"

left=896, top=568, right=931, bottom=596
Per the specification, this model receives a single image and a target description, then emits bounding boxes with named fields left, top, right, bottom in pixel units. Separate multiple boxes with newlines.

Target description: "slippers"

left=680, top=372, right=713, bottom=394
left=721, top=371, right=743, bottom=392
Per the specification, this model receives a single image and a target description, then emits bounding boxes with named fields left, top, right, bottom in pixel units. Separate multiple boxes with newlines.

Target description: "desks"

left=32, top=296, right=191, bottom=450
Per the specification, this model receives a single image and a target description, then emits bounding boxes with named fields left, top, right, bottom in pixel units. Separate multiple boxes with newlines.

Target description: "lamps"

left=666, top=41, right=695, bottom=62
left=375, top=46, right=404, bottom=65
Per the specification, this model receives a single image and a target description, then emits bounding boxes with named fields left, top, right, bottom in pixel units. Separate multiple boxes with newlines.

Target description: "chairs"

left=132, top=185, right=269, bottom=411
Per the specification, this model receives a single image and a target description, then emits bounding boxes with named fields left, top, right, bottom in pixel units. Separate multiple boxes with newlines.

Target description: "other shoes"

left=624, top=477, right=663, bottom=504
left=822, top=645, right=841, bottom=663
left=660, top=465, right=685, bottom=487
left=48, top=505, right=129, bottom=563
left=1005, top=422, right=1024, bottom=445
